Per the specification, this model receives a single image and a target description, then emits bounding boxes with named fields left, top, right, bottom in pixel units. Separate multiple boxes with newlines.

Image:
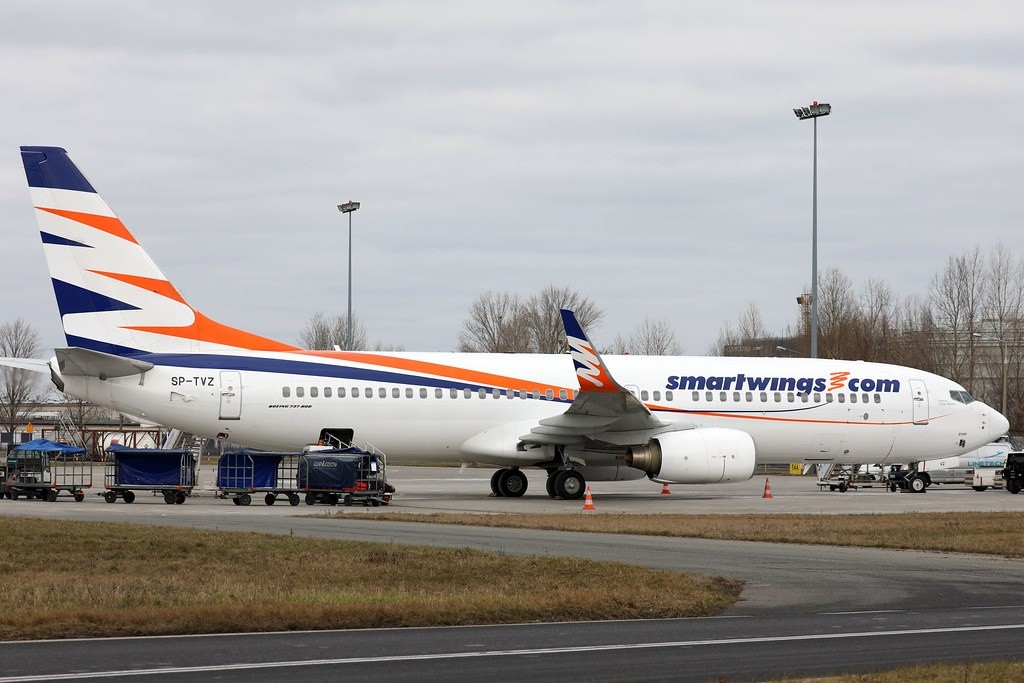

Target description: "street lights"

left=793, top=100, right=832, bottom=358
left=972, top=332, right=1007, bottom=418
left=336, top=200, right=360, bottom=350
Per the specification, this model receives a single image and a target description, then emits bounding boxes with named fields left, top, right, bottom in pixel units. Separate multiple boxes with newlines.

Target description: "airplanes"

left=0, top=146, right=1011, bottom=501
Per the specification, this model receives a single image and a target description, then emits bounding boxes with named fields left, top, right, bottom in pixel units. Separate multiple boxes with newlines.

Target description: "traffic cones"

left=659, top=483, right=672, bottom=495
left=762, top=477, right=774, bottom=499
left=582, top=484, right=596, bottom=510
